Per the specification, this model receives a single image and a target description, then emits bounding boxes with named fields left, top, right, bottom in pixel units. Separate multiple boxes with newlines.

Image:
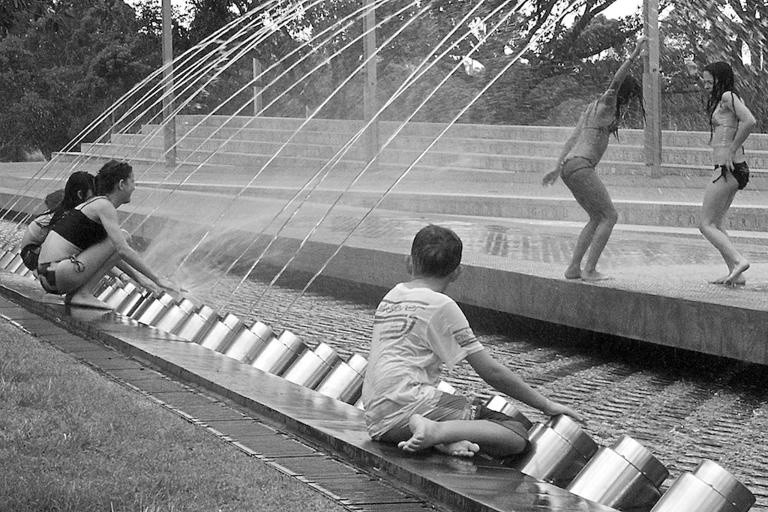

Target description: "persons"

left=700, top=61, right=756, bottom=286
left=38, top=157, right=189, bottom=311
left=48, top=170, right=132, bottom=288
left=21, top=189, right=66, bottom=277
left=542, top=37, right=648, bottom=279
left=362, top=224, right=588, bottom=459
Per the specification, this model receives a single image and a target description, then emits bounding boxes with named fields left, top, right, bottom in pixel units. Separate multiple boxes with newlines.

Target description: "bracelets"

left=119, top=273, right=124, bottom=278
left=628, top=57, right=634, bottom=62
